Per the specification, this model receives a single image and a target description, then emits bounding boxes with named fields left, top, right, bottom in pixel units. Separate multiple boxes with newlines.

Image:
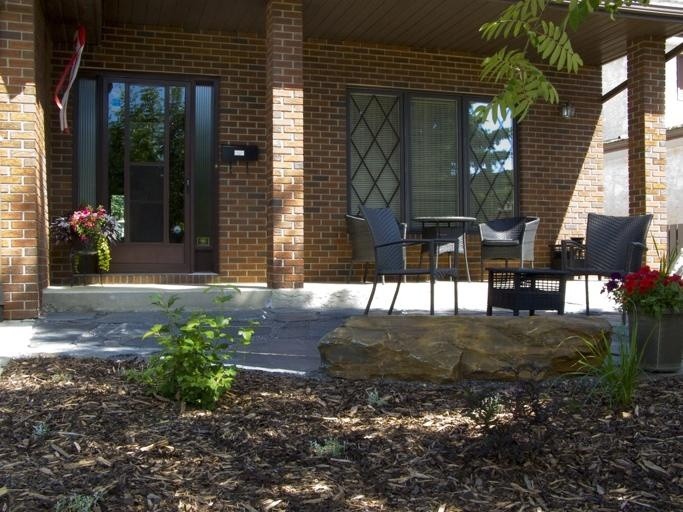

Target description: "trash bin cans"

left=548, top=244, right=573, bottom=280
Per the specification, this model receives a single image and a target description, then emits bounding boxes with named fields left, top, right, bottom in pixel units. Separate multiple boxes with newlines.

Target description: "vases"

left=623, top=315, right=682, bottom=374
left=76, top=251, right=98, bottom=275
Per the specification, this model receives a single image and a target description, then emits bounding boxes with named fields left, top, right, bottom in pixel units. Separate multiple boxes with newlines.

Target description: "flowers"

left=48, top=204, right=124, bottom=271
left=599, top=265, right=682, bottom=315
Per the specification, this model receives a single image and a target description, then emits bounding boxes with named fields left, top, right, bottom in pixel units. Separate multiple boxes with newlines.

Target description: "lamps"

left=560, top=99, right=577, bottom=121
